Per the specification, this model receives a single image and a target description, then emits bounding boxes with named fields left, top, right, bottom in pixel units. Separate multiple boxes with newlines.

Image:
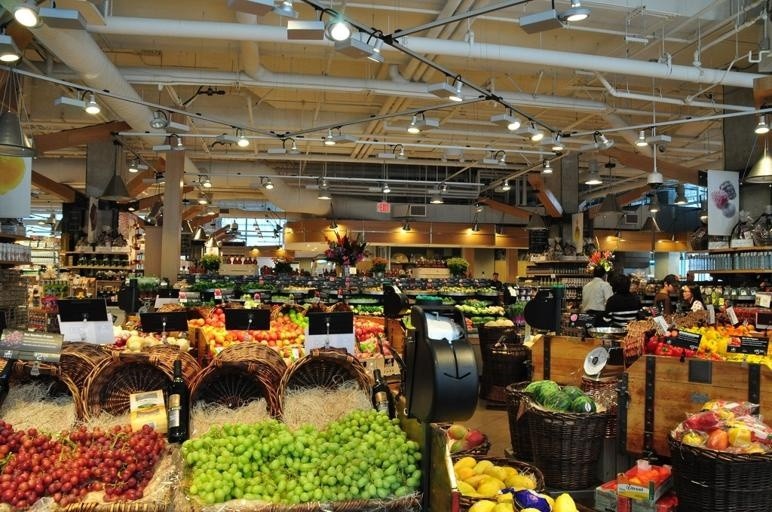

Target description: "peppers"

left=647, top=324, right=755, bottom=363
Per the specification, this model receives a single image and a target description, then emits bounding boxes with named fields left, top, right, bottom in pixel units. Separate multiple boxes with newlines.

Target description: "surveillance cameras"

left=646, top=171, right=663, bottom=189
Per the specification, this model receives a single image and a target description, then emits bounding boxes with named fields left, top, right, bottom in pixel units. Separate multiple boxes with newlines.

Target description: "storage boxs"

left=594, top=479, right=678, bottom=512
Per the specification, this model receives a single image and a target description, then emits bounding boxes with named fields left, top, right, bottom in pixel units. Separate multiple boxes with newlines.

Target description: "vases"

left=341, top=265, right=350, bottom=290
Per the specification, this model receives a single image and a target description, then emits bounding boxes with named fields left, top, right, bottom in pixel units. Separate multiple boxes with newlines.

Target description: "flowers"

left=320, top=229, right=371, bottom=267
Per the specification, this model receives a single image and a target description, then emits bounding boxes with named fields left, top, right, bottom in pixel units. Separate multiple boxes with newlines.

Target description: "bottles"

left=0, top=222, right=32, bottom=263
left=373, top=368, right=397, bottom=420
left=168, top=358, right=190, bottom=442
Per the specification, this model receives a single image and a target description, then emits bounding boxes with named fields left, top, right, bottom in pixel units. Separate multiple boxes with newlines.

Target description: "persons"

left=489, top=273, right=502, bottom=292
left=581, top=266, right=615, bottom=337
left=600, top=274, right=642, bottom=328
left=683, top=282, right=707, bottom=313
left=653, top=274, right=680, bottom=316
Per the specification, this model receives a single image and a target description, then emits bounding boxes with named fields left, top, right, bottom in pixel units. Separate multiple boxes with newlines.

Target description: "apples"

left=188, top=308, right=309, bottom=347
left=354, top=317, right=384, bottom=341
left=675, top=400, right=772, bottom=453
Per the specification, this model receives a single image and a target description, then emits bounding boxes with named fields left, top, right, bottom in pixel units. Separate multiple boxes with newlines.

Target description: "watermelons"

left=524, top=378, right=597, bottom=415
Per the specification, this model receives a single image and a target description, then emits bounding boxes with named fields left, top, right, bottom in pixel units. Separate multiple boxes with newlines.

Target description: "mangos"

left=440, top=424, right=579, bottom=512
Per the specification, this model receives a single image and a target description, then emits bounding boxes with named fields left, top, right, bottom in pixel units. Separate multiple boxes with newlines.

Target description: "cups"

left=637, top=458, right=649, bottom=472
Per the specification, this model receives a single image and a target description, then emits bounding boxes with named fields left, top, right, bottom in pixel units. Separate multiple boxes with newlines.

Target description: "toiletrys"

left=724, top=304, right=738, bottom=326
left=706, top=304, right=715, bottom=325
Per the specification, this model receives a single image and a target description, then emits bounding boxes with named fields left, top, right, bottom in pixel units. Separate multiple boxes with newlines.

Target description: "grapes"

left=179, top=409, right=423, bottom=505
left=0, top=416, right=166, bottom=511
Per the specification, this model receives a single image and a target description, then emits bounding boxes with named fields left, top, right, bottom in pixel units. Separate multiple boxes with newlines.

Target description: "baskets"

left=79, top=352, right=176, bottom=438
left=59, top=340, right=289, bottom=371
left=436, top=422, right=493, bottom=456
left=0, top=359, right=82, bottom=445
left=505, top=380, right=566, bottom=463
left=522, top=395, right=612, bottom=491
left=277, top=347, right=374, bottom=426
left=452, top=454, right=547, bottom=512
left=187, top=359, right=277, bottom=432
left=666, top=429, right=772, bottom=512
left=156, top=302, right=352, bottom=319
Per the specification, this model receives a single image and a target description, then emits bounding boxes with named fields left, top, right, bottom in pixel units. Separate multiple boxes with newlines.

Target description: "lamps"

left=317, top=178, right=333, bottom=201
left=12, top=0, right=45, bottom=30
left=406, top=115, right=421, bottom=135
left=394, top=145, right=408, bottom=162
left=744, top=155, right=772, bottom=185
left=128, top=156, right=214, bottom=206
left=286, top=140, right=301, bottom=156
left=399, top=222, right=416, bottom=233
left=272, top=1, right=299, bottom=20
left=382, top=182, right=391, bottom=195
left=540, top=159, right=554, bottom=176
left=504, top=109, right=522, bottom=132
left=752, top=116, right=771, bottom=136
left=325, top=17, right=351, bottom=43
left=447, top=80, right=465, bottom=103
left=323, top=129, right=336, bottom=146
left=364, top=38, right=387, bottom=65
left=84, top=94, right=102, bottom=116
left=528, top=122, right=545, bottom=143
left=237, top=129, right=250, bottom=147
left=148, top=109, right=168, bottom=130
left=0, top=42, right=22, bottom=63
left=466, top=223, right=484, bottom=236
left=583, top=168, right=604, bottom=187
left=168, top=133, right=187, bottom=152
left=598, top=133, right=615, bottom=152
left=429, top=183, right=447, bottom=206
left=633, top=130, right=648, bottom=148
left=261, top=177, right=274, bottom=191
left=550, top=133, right=565, bottom=154
left=648, top=186, right=688, bottom=214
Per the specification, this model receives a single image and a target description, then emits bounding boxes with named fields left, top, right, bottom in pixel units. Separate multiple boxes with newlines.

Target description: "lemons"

left=125, top=334, right=190, bottom=354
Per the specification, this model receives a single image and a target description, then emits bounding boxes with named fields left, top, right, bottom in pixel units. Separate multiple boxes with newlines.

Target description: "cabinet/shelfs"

left=517, top=260, right=608, bottom=325
left=0, top=232, right=146, bottom=333
left=683, top=245, right=772, bottom=315
